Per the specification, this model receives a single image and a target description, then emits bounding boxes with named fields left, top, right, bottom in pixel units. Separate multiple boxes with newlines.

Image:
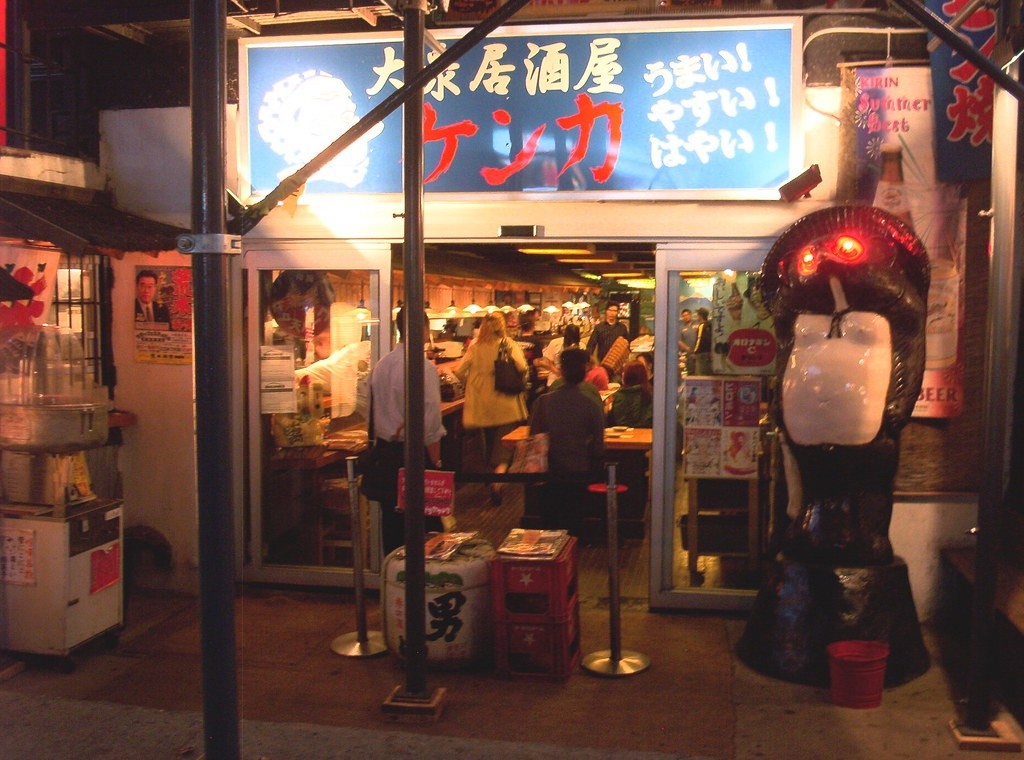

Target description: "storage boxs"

left=487, top=535, right=579, bottom=623
left=491, top=603, right=582, bottom=686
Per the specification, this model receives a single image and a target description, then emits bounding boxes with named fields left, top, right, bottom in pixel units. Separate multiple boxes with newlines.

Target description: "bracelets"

left=431, top=460, right=442, bottom=467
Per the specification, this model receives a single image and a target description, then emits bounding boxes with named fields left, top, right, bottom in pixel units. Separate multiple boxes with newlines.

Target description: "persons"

left=585, top=301, right=629, bottom=361
left=513, top=310, right=543, bottom=392
left=635, top=353, right=654, bottom=384
left=543, top=324, right=586, bottom=388
left=691, top=308, right=712, bottom=375
left=606, top=361, right=655, bottom=428
left=371, top=308, right=446, bottom=558
left=584, top=350, right=608, bottom=391
left=530, top=348, right=605, bottom=541
left=457, top=312, right=529, bottom=505
left=136, top=270, right=169, bottom=323
left=679, top=309, right=698, bottom=375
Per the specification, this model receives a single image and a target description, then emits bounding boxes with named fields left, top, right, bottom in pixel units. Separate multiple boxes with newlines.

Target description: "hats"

left=695, top=308, right=709, bottom=320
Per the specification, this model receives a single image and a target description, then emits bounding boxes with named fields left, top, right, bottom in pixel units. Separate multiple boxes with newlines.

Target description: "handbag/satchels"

left=494, top=336, right=524, bottom=394
left=359, top=446, right=381, bottom=502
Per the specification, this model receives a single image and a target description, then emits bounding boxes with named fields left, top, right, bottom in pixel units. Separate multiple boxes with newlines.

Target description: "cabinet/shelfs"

left=0, top=497, right=126, bottom=675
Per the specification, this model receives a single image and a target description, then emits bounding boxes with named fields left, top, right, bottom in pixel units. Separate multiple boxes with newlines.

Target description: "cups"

left=922, top=262, right=960, bottom=370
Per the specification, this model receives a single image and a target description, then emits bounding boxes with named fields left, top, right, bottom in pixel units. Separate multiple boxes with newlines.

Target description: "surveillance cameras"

left=779, top=165, right=822, bottom=203
left=226, top=190, right=246, bottom=218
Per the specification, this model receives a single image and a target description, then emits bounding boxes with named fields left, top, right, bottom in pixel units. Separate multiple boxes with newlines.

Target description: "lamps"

left=423, top=287, right=434, bottom=318
left=562, top=292, right=575, bottom=308
left=575, top=293, right=590, bottom=309
left=484, top=290, right=500, bottom=313
left=439, top=288, right=461, bottom=316
left=516, top=290, right=535, bottom=313
left=500, top=290, right=516, bottom=314
left=463, top=289, right=483, bottom=314
left=392, top=286, right=402, bottom=314
left=351, top=281, right=372, bottom=321
left=543, top=292, right=560, bottom=313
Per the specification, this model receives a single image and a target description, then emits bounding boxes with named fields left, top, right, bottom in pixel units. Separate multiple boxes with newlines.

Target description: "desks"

left=500, top=426, right=652, bottom=450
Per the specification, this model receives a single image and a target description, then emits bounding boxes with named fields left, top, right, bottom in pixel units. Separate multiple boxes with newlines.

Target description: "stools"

left=588, top=484, right=630, bottom=546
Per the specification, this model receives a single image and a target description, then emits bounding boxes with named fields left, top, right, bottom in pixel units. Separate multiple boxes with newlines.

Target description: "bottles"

left=872, top=144, right=916, bottom=230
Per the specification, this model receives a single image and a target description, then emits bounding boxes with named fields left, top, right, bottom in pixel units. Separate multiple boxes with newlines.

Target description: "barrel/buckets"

left=827, top=640, right=889, bottom=708
